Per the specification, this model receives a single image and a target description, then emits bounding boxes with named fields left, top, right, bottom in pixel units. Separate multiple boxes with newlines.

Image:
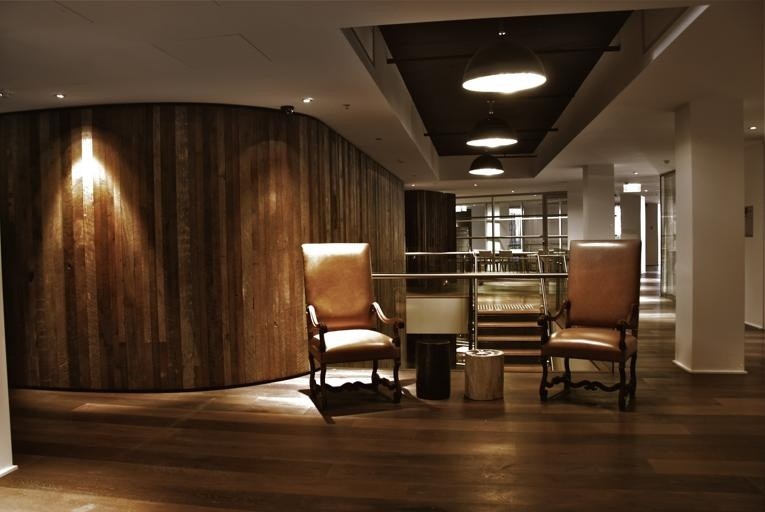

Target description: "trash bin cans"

left=416, top=340, right=451, bottom=400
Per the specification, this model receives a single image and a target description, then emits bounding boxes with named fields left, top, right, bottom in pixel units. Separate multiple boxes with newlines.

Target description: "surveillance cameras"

left=280, top=105, right=294, bottom=117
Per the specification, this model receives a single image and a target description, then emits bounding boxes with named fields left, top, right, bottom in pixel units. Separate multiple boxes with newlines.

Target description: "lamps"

left=460, top=18, right=549, bottom=177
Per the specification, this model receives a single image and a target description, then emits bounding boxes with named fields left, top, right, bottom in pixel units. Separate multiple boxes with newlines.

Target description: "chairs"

left=539, top=238, right=642, bottom=411
left=472, top=247, right=567, bottom=272
left=300, top=241, right=404, bottom=411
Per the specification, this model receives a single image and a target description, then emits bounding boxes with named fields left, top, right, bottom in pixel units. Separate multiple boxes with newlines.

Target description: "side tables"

left=415, top=341, right=505, bottom=401
